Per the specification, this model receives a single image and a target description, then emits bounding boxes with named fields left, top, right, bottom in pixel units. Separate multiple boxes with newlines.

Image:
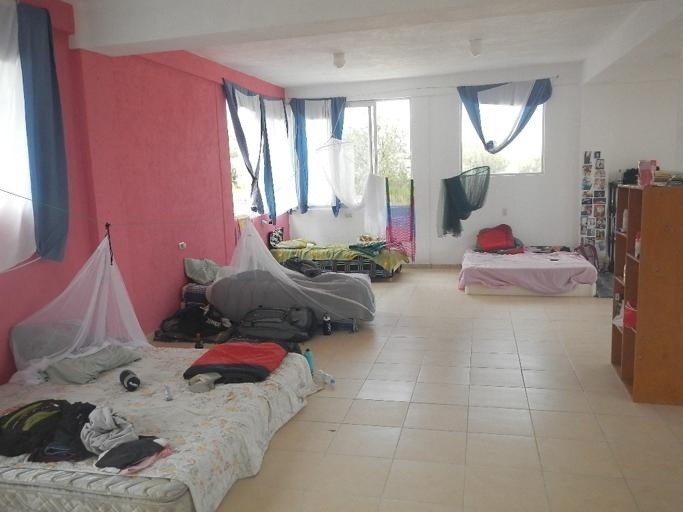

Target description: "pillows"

left=183, top=257, right=222, bottom=285
left=12, top=312, right=91, bottom=374
left=50, top=340, right=141, bottom=384
left=275, top=239, right=307, bottom=249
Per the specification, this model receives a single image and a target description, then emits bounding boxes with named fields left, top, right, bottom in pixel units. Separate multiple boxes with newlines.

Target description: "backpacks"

left=0, top=399, right=70, bottom=458
left=237, top=307, right=313, bottom=339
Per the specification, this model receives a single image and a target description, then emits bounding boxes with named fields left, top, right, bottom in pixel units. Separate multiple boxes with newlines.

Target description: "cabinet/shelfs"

left=609, top=186, right=683, bottom=406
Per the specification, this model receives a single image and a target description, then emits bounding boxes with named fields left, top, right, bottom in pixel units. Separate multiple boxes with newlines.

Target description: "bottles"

left=163, top=383, right=171, bottom=401
left=120, top=369, right=140, bottom=390
left=303, top=348, right=335, bottom=385
left=322, top=313, right=331, bottom=336
left=637, top=161, right=651, bottom=186
left=194, top=333, right=203, bottom=349
left=616, top=169, right=622, bottom=186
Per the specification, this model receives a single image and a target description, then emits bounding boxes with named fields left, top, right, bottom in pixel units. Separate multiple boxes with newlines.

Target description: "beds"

left=181, top=266, right=376, bottom=334
left=461, top=246, right=597, bottom=298
left=0, top=343, right=314, bottom=512
left=266, top=239, right=405, bottom=278
